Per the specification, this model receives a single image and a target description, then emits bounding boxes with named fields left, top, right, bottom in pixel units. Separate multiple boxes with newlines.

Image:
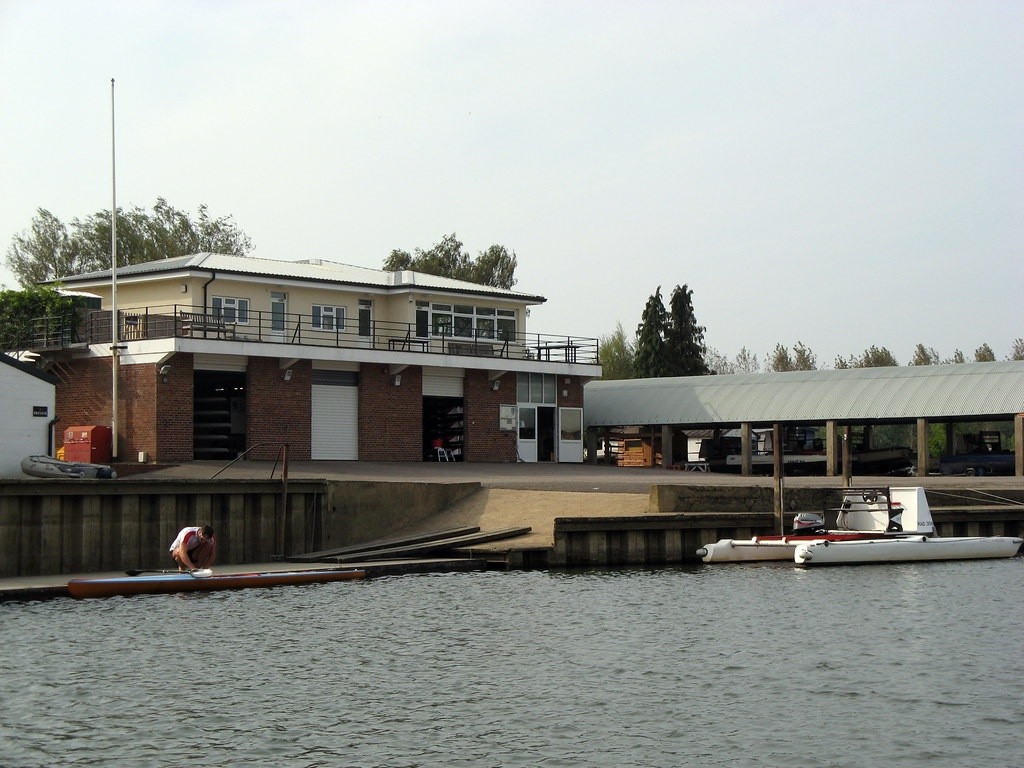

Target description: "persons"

left=169, top=525, right=215, bottom=574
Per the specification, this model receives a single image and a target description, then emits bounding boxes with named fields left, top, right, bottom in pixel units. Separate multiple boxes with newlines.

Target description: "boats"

left=938, top=433, right=1016, bottom=478
left=726, top=427, right=912, bottom=476
left=695, top=485, right=1024, bottom=569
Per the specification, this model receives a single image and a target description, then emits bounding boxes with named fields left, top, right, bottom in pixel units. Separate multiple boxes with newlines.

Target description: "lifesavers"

left=20, top=454, right=119, bottom=480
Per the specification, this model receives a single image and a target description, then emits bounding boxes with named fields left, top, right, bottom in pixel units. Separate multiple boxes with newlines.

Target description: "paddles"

left=125, top=568, right=213, bottom=578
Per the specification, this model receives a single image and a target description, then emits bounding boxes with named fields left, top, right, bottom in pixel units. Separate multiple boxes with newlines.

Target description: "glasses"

left=201, top=532, right=208, bottom=542
